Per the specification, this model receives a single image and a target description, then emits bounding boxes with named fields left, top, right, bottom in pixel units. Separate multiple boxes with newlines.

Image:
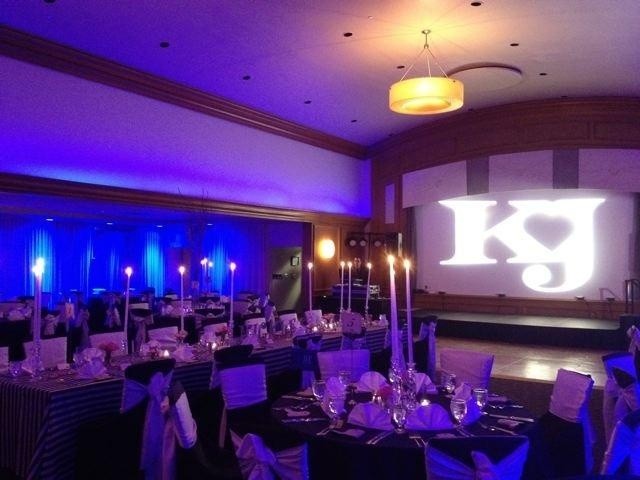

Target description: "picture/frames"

left=291, top=256, right=300, bottom=266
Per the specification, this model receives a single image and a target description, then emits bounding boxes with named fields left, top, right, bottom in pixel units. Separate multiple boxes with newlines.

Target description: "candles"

left=306, top=253, right=416, bottom=364
left=32, top=256, right=45, bottom=339
left=123, top=259, right=237, bottom=331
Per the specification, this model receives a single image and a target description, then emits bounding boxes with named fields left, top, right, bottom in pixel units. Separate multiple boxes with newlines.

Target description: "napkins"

left=405, top=404, right=453, bottom=433
left=452, top=380, right=473, bottom=414
left=402, top=371, right=439, bottom=396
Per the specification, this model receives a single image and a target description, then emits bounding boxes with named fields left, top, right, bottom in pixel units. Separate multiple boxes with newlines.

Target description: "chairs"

left=400, top=317, right=437, bottom=377
left=600, top=412, right=637, bottom=478
left=601, top=352, right=637, bottom=447
left=423, top=435, right=529, bottom=480
left=0, top=290, right=411, bottom=479
left=549, top=367, right=597, bottom=476
left=440, top=349, right=495, bottom=390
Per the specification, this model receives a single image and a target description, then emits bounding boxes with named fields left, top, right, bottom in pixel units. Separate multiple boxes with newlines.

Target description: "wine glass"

left=472, top=388, right=489, bottom=414
left=311, top=379, right=326, bottom=406
left=450, top=398, right=467, bottom=430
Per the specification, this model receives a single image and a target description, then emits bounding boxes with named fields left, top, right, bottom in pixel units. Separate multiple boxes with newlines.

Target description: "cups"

left=337, top=370, right=352, bottom=386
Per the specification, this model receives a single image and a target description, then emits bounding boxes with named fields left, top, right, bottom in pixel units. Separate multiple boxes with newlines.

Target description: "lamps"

left=386, top=26, right=466, bottom=116
